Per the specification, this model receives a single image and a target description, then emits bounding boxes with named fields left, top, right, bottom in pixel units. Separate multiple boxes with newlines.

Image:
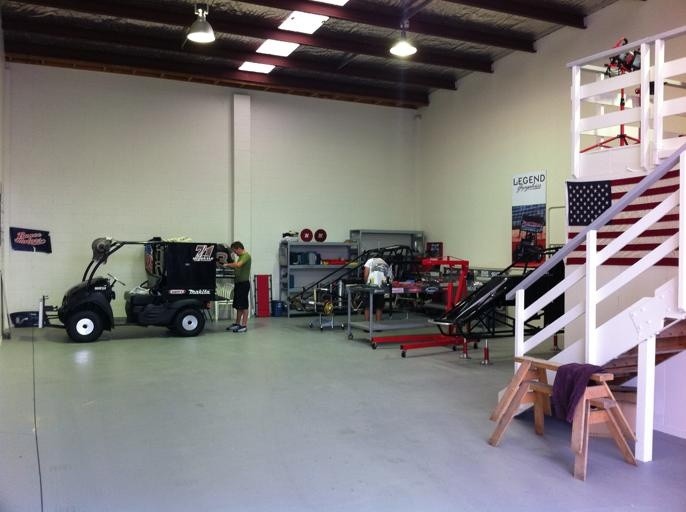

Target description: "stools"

left=215, top=299, right=235, bottom=322
left=489, top=356, right=638, bottom=481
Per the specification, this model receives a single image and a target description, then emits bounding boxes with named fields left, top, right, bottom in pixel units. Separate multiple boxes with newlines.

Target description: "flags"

left=11, top=226, right=53, bottom=253
left=566, top=177, right=680, bottom=267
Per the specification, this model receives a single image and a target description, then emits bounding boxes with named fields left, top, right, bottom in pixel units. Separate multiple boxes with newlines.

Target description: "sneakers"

left=233, top=325, right=248, bottom=332
left=226, top=323, right=242, bottom=330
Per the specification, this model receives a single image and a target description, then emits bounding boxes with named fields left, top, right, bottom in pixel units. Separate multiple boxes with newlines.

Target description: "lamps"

left=390, top=17, right=417, bottom=58
left=187, top=0, right=217, bottom=45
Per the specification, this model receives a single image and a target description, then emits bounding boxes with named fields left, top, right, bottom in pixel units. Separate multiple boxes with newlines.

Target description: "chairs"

left=124, top=273, right=164, bottom=306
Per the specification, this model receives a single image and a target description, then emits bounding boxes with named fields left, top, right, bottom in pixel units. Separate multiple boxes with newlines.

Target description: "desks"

left=346, top=281, right=479, bottom=343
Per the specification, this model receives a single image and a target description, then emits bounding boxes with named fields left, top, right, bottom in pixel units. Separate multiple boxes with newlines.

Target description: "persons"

left=222, top=241, right=251, bottom=332
left=362, top=255, right=392, bottom=333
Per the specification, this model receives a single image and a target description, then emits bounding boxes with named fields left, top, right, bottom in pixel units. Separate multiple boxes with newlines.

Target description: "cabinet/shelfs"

left=278, top=241, right=360, bottom=318
left=350, top=229, right=424, bottom=277
